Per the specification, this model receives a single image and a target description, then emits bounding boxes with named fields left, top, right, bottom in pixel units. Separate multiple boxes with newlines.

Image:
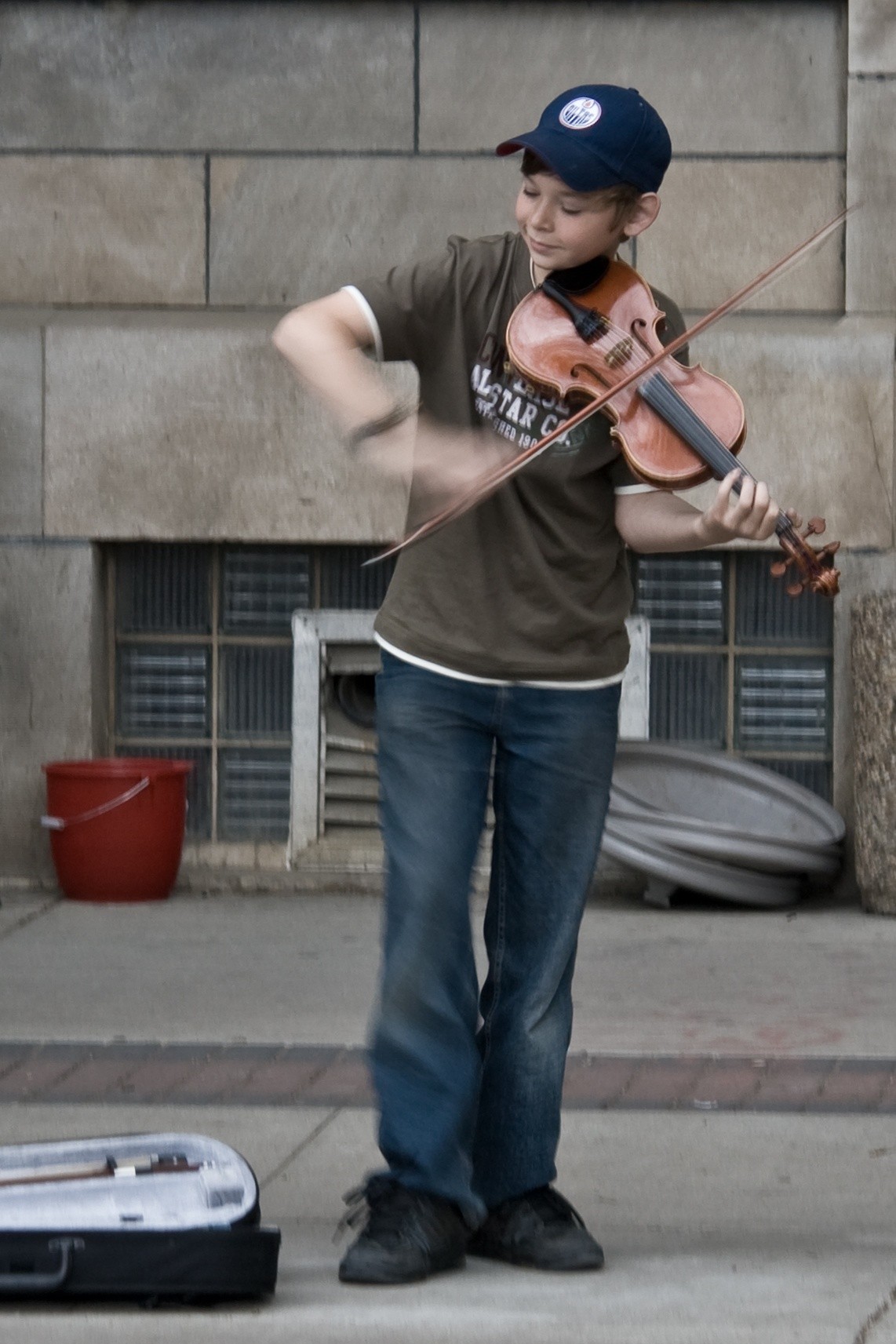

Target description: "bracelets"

left=341, top=403, right=414, bottom=447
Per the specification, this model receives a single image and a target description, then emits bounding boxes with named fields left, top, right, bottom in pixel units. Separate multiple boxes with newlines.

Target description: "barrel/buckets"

left=41, top=757, right=194, bottom=902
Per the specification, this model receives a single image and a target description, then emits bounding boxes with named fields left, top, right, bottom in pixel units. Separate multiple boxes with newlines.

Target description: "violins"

left=505, top=255, right=842, bottom=601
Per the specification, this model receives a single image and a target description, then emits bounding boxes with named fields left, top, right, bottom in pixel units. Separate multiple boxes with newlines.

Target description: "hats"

left=496, top=82, right=671, bottom=195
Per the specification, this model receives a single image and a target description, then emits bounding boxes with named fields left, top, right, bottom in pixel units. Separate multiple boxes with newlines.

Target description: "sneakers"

left=467, top=1187, right=607, bottom=1274
left=335, top=1174, right=463, bottom=1287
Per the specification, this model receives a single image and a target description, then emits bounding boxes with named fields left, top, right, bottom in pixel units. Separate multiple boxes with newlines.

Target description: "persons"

left=271, top=84, right=780, bottom=1284
